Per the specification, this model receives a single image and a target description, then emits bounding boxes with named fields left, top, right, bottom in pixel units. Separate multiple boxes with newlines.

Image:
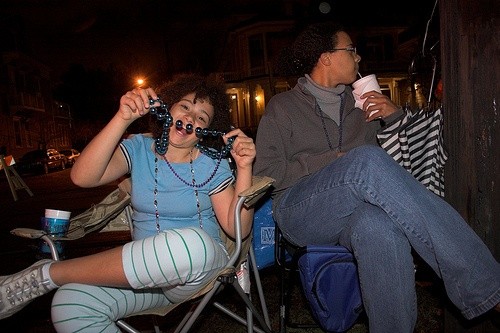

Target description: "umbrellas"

left=378, top=50, right=446, bottom=198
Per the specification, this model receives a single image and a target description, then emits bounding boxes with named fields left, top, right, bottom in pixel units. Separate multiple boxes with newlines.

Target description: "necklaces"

left=149, top=97, right=235, bottom=234
left=315, top=95, right=344, bottom=150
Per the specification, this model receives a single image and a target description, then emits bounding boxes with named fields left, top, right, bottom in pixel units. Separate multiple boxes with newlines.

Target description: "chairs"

left=272, top=221, right=418, bottom=333
left=9, top=176, right=275, bottom=333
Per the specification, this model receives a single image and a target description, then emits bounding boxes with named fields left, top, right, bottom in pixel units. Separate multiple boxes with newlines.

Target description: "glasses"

left=321, top=45, right=358, bottom=54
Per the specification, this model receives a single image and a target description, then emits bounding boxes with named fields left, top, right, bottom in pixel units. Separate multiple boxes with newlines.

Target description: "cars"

left=22, top=148, right=67, bottom=175
left=58, top=149, right=80, bottom=168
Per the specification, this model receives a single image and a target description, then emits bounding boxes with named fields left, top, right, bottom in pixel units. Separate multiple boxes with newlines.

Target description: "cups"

left=43, top=209, right=72, bottom=253
left=351, top=73, right=382, bottom=120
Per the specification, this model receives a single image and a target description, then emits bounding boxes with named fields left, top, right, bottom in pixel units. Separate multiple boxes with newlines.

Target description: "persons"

left=253, top=26, right=500, bottom=333
left=0, top=75, right=256, bottom=333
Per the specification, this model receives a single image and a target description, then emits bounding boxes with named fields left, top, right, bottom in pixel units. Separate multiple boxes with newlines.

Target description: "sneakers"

left=0, top=258, right=56, bottom=318
